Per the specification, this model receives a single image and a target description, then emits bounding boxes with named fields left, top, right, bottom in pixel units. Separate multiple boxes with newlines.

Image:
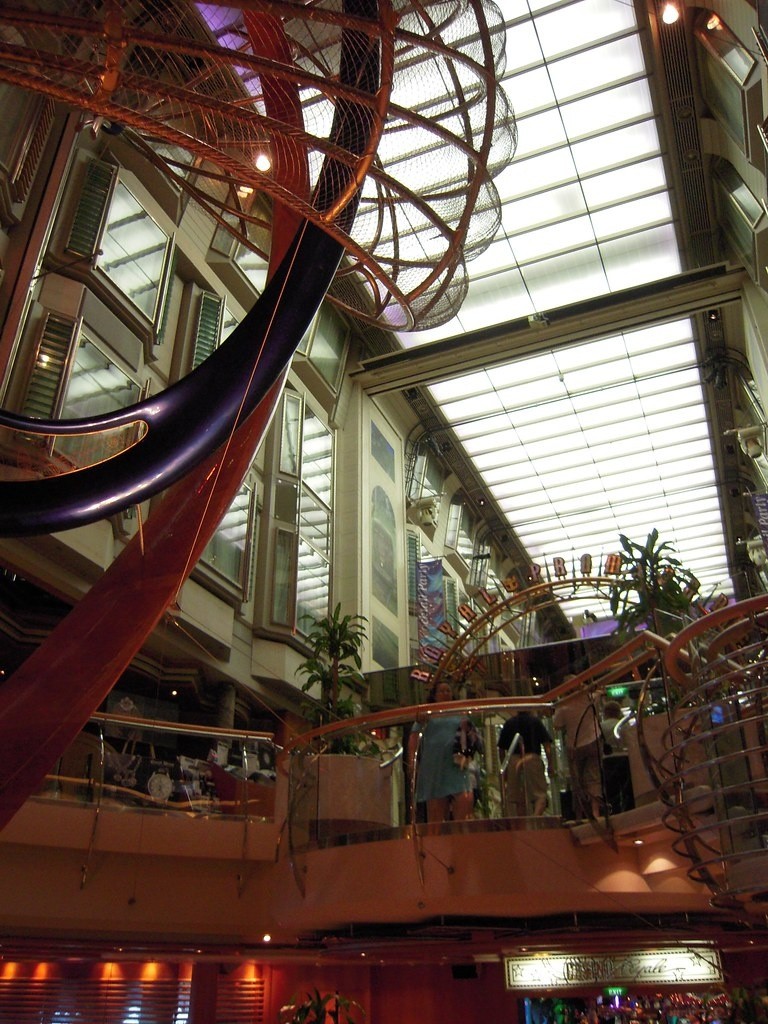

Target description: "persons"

left=496, top=706, right=554, bottom=816
left=600, top=700, right=635, bottom=816
left=408, top=680, right=474, bottom=835
left=554, top=674, right=603, bottom=826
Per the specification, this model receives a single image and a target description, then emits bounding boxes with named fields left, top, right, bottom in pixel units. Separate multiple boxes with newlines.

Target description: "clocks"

left=149, top=775, right=173, bottom=799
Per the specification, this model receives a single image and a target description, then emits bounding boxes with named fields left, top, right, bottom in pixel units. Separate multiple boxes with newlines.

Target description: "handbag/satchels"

left=603, top=742, right=613, bottom=754
